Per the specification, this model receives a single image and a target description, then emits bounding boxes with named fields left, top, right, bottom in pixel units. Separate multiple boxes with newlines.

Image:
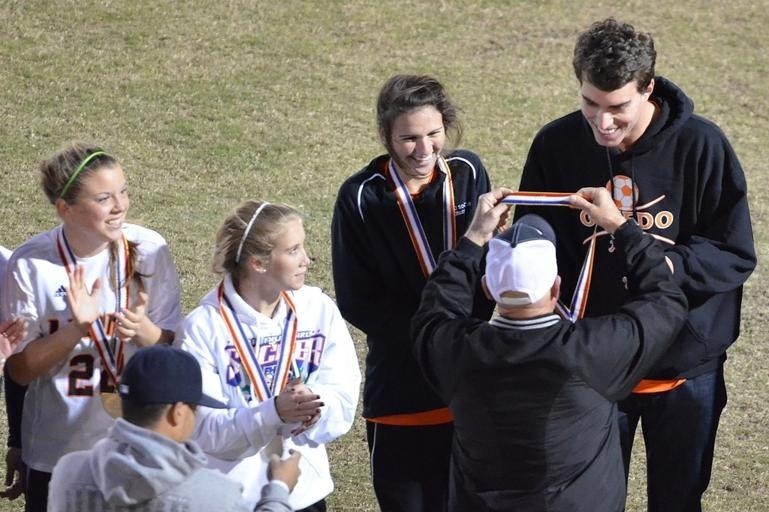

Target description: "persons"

left=185, top=195, right=361, bottom=511
left=0, top=317, right=29, bottom=374
left=513, top=15, right=756, bottom=511
left=0, top=140, right=185, bottom=511
left=0, top=245, right=29, bottom=501
left=407, top=181, right=687, bottom=512
left=46, top=343, right=303, bottom=511
left=327, top=75, right=493, bottom=511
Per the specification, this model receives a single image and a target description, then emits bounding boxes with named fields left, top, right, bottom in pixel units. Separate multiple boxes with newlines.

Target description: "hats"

left=116, top=343, right=230, bottom=411
left=483, top=212, right=561, bottom=308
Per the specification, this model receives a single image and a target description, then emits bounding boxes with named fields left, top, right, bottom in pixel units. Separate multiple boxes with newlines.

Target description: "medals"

left=101, top=378, right=123, bottom=418
left=264, top=434, right=283, bottom=458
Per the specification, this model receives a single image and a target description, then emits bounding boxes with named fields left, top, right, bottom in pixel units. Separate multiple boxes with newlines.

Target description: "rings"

left=2, top=332, right=8, bottom=337
left=296, top=402, right=301, bottom=410
